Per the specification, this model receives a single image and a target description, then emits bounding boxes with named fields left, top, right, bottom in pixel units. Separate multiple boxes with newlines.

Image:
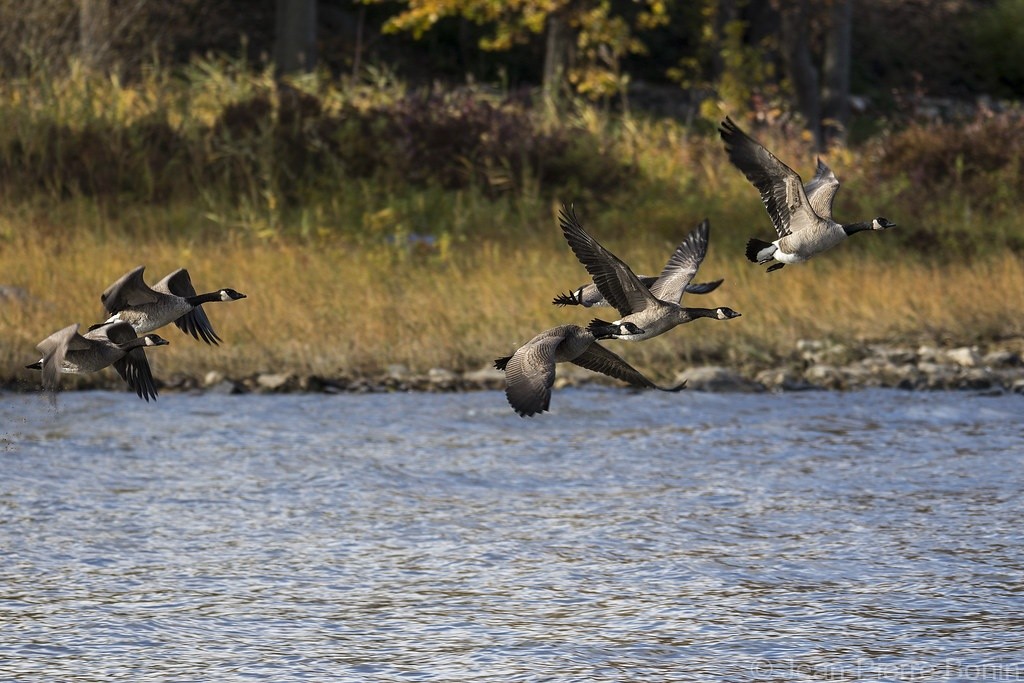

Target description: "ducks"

left=491, top=200, right=745, bottom=420
left=24, top=264, right=249, bottom=405
left=714, top=115, right=899, bottom=276
